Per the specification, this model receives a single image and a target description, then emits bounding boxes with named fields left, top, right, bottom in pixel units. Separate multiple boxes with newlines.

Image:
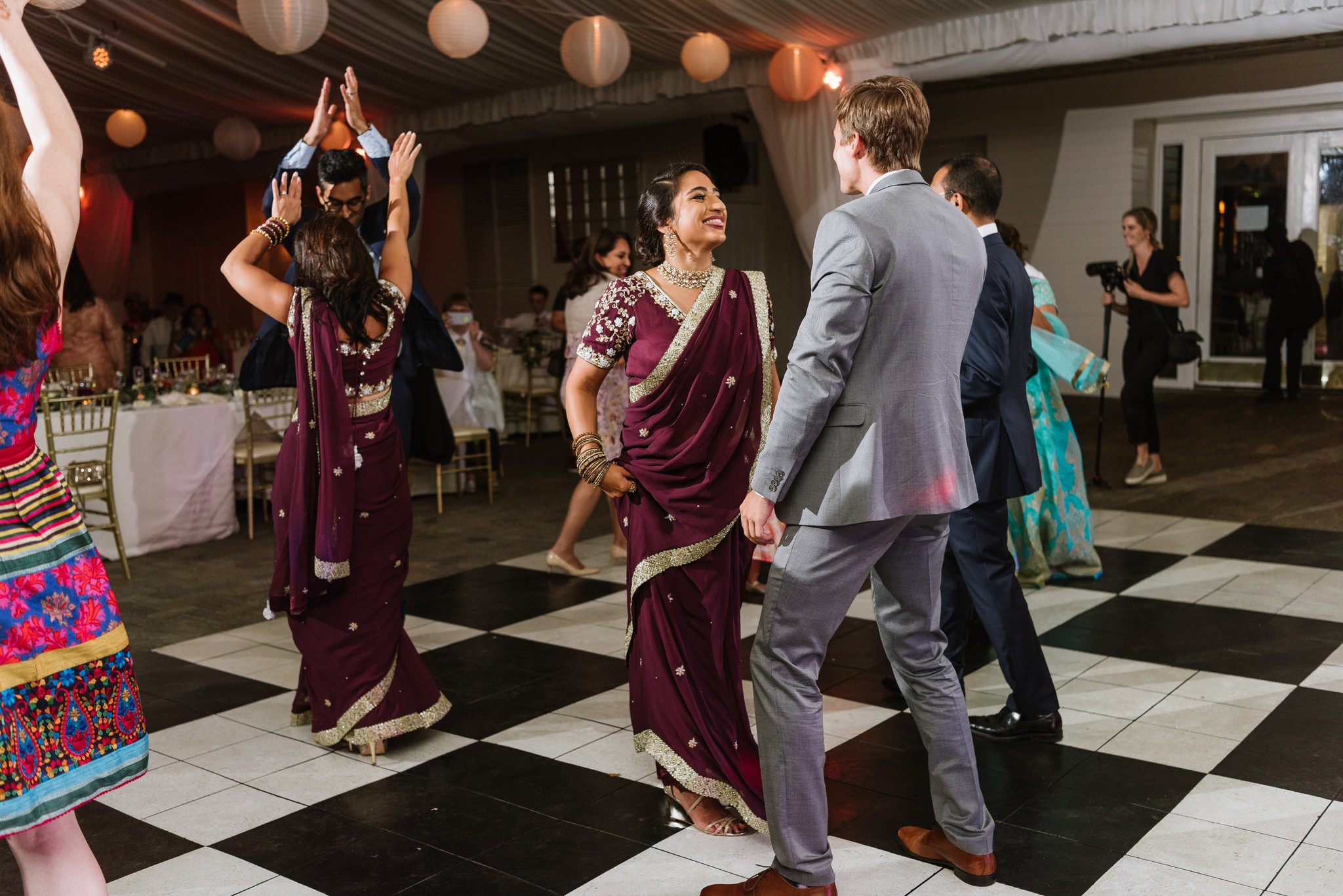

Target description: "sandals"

left=662, top=783, right=748, bottom=837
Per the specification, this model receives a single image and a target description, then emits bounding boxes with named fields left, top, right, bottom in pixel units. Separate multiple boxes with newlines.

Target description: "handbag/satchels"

left=1166, top=316, right=1203, bottom=365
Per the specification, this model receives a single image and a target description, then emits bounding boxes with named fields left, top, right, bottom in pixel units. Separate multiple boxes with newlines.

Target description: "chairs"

left=499, top=338, right=567, bottom=448
left=409, top=427, right=493, bottom=516
left=41, top=363, right=96, bottom=396
left=151, top=355, right=213, bottom=379
left=235, top=385, right=298, bottom=540
left=37, top=387, right=134, bottom=583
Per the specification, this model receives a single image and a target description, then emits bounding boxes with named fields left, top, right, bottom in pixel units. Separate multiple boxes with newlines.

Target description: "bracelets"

left=357, top=122, right=373, bottom=137
left=572, top=432, right=612, bottom=489
left=250, top=216, right=290, bottom=252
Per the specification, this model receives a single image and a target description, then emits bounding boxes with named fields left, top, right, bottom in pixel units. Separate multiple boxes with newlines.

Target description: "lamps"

left=821, top=52, right=844, bottom=91
left=213, top=116, right=261, bottom=159
left=83, top=43, right=111, bottom=70
left=680, top=33, right=730, bottom=83
left=767, top=45, right=825, bottom=102
left=105, top=108, right=146, bottom=146
left=561, top=16, right=631, bottom=90
left=319, top=121, right=351, bottom=153
left=236, top=0, right=329, bottom=54
left=427, top=0, right=490, bottom=59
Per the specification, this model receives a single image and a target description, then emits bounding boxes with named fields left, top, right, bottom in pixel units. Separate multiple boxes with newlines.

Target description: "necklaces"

left=656, top=257, right=713, bottom=290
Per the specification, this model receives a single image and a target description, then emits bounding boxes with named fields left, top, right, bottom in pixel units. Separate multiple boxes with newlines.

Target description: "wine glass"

left=40, top=358, right=237, bottom=404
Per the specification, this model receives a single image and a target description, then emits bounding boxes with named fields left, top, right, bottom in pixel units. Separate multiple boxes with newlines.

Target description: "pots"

left=67, top=460, right=104, bottom=487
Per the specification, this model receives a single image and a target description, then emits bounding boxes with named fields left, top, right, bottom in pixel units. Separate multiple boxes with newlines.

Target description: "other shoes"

left=467, top=480, right=476, bottom=493
left=1124, top=458, right=1156, bottom=485
left=1141, top=467, right=1169, bottom=485
left=746, top=580, right=767, bottom=594
left=491, top=478, right=500, bottom=492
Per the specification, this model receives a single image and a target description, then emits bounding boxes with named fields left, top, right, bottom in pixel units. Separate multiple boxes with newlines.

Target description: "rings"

left=628, top=483, right=636, bottom=493
left=323, top=99, right=330, bottom=103
left=347, top=91, right=355, bottom=95
left=280, top=190, right=288, bottom=196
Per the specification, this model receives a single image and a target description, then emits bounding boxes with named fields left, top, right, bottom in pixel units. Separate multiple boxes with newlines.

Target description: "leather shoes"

left=897, top=825, right=996, bottom=887
left=966, top=705, right=1063, bottom=742
left=700, top=866, right=838, bottom=896
left=882, top=677, right=967, bottom=708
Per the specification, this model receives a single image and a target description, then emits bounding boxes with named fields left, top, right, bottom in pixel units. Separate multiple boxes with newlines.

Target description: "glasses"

left=318, top=190, right=367, bottom=212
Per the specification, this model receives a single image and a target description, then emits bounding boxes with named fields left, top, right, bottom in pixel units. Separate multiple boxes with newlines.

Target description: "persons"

left=563, top=162, right=781, bottom=838
left=1253, top=238, right=1325, bottom=404
left=698, top=72, right=999, bottom=896
left=992, top=217, right=1110, bottom=588
left=220, top=128, right=452, bottom=768
left=0, top=0, right=153, bottom=896
left=240, top=65, right=465, bottom=469
left=547, top=223, right=637, bottom=576
left=56, top=232, right=593, bottom=495
left=882, top=153, right=1065, bottom=744
left=1100, top=205, right=1192, bottom=487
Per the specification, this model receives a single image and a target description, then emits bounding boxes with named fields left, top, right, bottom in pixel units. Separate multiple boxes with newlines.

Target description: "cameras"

left=1085, top=260, right=1125, bottom=294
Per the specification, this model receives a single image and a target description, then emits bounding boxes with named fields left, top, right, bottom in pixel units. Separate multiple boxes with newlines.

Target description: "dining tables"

left=35, top=389, right=297, bottom=558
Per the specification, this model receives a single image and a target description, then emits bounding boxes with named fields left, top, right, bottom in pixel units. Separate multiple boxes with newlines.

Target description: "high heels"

left=546, top=550, right=600, bottom=576
left=361, top=738, right=388, bottom=763
left=611, top=544, right=627, bottom=564
